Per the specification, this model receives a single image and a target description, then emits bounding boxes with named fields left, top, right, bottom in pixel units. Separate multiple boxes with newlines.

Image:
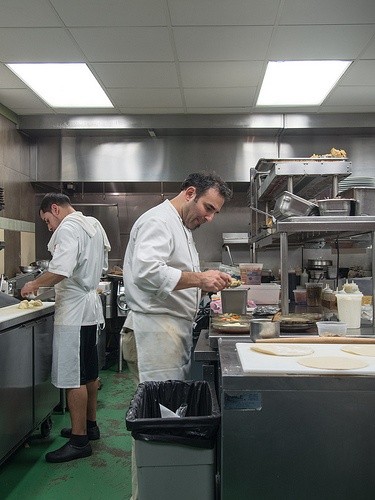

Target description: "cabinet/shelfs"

left=0, top=313, right=70, bottom=463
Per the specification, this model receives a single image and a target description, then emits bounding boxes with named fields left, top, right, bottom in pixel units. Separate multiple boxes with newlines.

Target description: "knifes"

left=34, top=288, right=55, bottom=300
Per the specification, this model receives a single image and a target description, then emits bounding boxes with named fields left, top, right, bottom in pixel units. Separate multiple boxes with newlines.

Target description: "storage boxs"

left=216, top=282, right=279, bottom=304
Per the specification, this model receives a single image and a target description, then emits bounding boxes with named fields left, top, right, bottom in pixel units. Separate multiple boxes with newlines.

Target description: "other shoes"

left=60, top=428, right=100, bottom=440
left=45, top=440, right=92, bottom=463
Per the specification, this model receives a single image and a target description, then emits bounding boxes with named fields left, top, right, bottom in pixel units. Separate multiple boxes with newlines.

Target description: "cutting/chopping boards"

left=236, top=342, right=375, bottom=376
left=0, top=302, right=55, bottom=322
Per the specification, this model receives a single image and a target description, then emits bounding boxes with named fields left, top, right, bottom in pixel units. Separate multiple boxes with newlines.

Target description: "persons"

left=21, top=194, right=111, bottom=465
left=123, top=167, right=233, bottom=390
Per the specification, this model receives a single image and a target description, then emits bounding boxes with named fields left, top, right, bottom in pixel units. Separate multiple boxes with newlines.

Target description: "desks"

left=219, top=337, right=375, bottom=500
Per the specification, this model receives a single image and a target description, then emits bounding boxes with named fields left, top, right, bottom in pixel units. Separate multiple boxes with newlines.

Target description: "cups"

left=239, top=263, right=263, bottom=285
left=304, top=283, right=323, bottom=307
left=334, top=293, right=364, bottom=329
left=292, top=290, right=307, bottom=305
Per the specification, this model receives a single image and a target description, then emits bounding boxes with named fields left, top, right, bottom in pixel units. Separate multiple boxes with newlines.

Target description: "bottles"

left=288, top=268, right=297, bottom=298
left=322, top=279, right=358, bottom=302
left=300, top=269, right=309, bottom=286
left=2, top=280, right=8, bottom=295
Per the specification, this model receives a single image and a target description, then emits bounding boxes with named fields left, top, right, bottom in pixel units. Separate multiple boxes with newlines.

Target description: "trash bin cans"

left=127, top=380, right=222, bottom=500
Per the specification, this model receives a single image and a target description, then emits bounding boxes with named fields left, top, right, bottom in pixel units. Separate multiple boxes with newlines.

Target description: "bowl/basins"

left=250, top=319, right=280, bottom=342
left=19, top=266, right=38, bottom=273
left=316, top=321, right=349, bottom=337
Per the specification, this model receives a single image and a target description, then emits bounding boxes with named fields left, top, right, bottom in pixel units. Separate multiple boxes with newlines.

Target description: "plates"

left=280, top=323, right=311, bottom=332
